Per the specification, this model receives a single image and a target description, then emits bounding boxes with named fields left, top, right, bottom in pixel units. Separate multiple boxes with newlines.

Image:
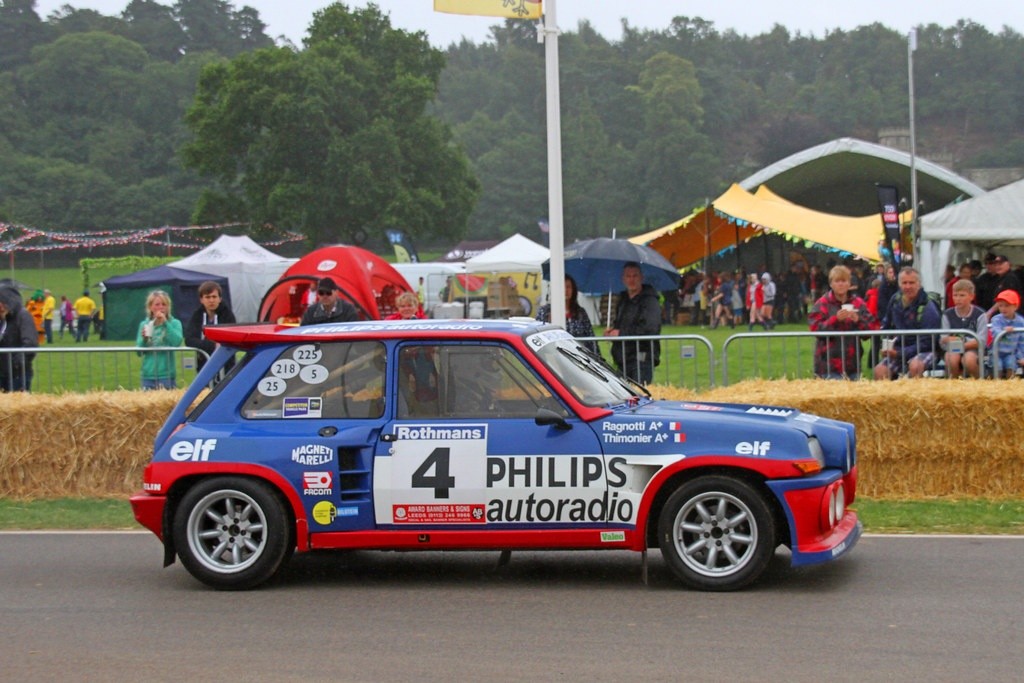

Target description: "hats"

left=317, top=278, right=335, bottom=290
left=992, top=255, right=1007, bottom=263
left=994, top=290, right=1019, bottom=306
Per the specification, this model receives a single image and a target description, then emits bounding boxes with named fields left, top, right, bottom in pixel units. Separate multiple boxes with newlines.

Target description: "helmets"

left=440, top=338, right=504, bottom=393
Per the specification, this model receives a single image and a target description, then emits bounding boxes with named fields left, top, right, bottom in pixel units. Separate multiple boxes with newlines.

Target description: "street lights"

left=432, top=1, right=567, bottom=329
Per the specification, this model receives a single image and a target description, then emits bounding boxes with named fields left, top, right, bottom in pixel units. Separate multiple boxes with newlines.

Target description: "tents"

left=100, top=231, right=550, bottom=341
left=625, top=181, right=912, bottom=326
left=918, top=179, right=1024, bottom=303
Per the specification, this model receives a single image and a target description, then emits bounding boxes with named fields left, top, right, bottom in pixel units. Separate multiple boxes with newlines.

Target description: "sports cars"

left=129, top=318, right=863, bottom=592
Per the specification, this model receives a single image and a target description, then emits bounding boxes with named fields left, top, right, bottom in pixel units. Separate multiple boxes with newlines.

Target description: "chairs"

left=391, top=363, right=439, bottom=418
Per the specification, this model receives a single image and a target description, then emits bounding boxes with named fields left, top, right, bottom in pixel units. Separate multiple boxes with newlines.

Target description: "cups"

left=144, top=324, right=154, bottom=337
left=841, top=303, right=854, bottom=317
left=882, top=338, right=894, bottom=351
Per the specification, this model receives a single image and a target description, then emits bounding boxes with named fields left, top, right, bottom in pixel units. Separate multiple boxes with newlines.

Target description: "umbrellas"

left=540, top=226, right=682, bottom=335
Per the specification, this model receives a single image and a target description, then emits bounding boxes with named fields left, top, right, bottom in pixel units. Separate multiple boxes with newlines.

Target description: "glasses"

left=319, top=290, right=331, bottom=296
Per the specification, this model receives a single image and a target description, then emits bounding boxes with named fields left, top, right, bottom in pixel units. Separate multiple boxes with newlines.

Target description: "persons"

left=384, top=293, right=427, bottom=320
left=662, top=250, right=1023, bottom=380
left=184, top=281, right=235, bottom=389
left=438, top=278, right=452, bottom=302
left=136, top=290, right=184, bottom=391
left=0, top=277, right=40, bottom=395
left=538, top=273, right=603, bottom=356
left=415, top=276, right=424, bottom=303
left=446, top=346, right=504, bottom=417
left=603, top=262, right=662, bottom=385
left=25, top=289, right=106, bottom=345
left=300, top=277, right=365, bottom=328
